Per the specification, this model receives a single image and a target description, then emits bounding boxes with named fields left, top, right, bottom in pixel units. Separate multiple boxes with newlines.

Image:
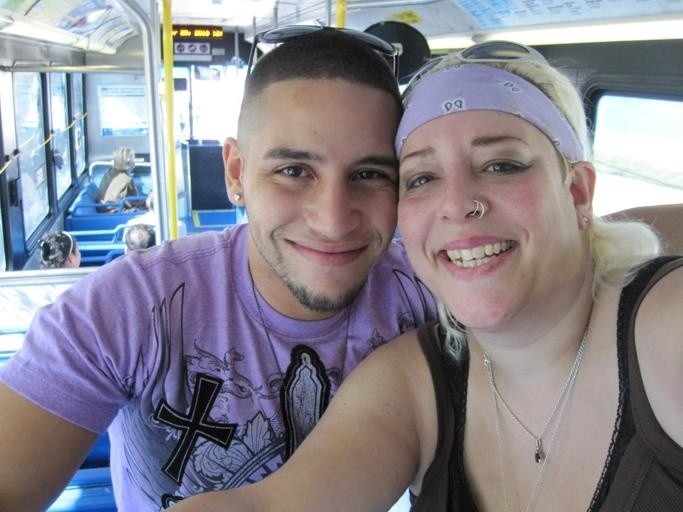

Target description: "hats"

left=36, top=230, right=72, bottom=269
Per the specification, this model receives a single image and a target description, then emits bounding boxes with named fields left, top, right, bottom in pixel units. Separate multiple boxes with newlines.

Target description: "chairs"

left=0, top=160, right=152, bottom=511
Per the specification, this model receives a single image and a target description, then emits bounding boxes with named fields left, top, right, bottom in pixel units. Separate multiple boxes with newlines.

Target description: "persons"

left=121, top=191, right=154, bottom=241
left=36, top=229, right=81, bottom=272
left=94, top=145, right=145, bottom=214
left=163, top=39, right=682, bottom=510
left=0, top=16, right=457, bottom=512
left=123, top=223, right=156, bottom=255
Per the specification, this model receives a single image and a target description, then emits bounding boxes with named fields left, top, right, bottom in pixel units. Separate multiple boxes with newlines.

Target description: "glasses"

left=400, top=40, right=552, bottom=102
left=245, top=24, right=397, bottom=84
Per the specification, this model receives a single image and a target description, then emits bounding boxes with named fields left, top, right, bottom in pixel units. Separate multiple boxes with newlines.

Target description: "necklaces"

left=480, top=363, right=582, bottom=511
left=475, top=326, right=609, bottom=466
